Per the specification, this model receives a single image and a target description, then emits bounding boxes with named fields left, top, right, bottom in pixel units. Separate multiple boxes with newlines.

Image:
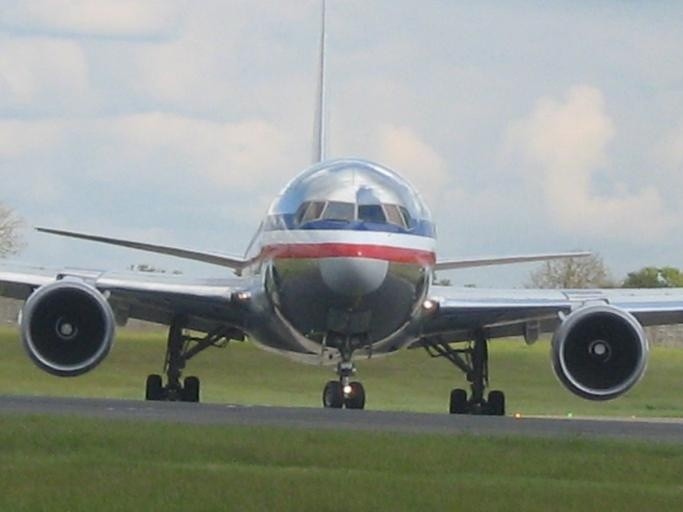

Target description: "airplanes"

left=0, top=0, right=683, bottom=417
